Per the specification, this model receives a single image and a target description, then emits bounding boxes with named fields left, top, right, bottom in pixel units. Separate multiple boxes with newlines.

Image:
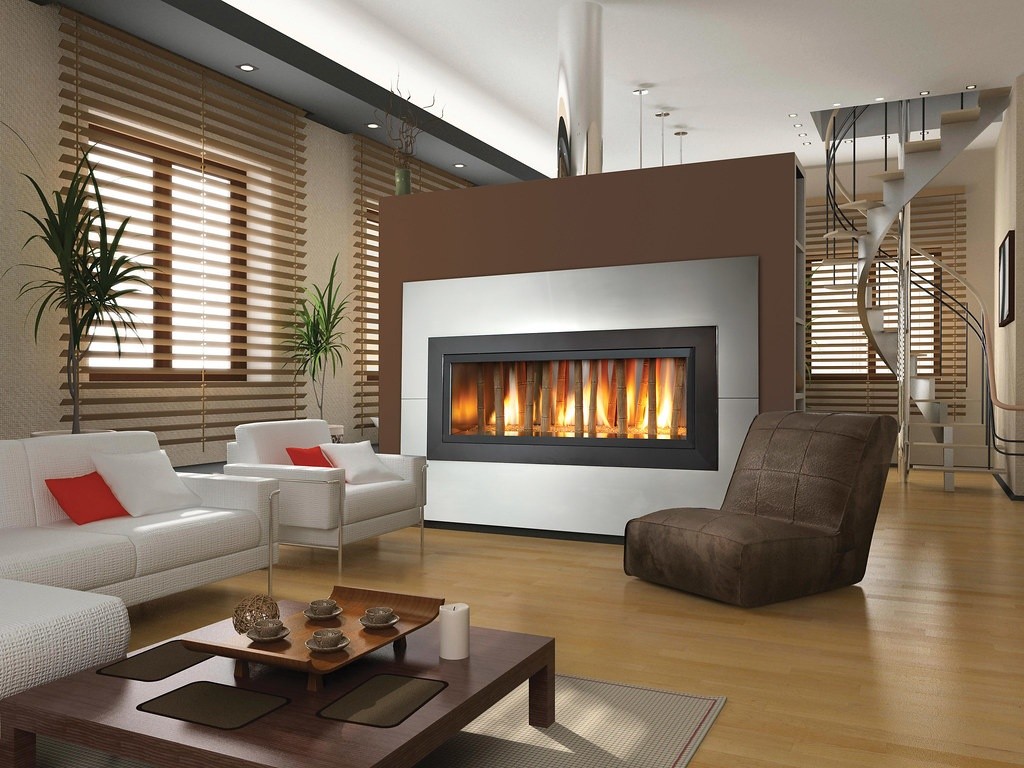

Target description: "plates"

left=246, top=628, right=291, bottom=643
left=305, top=637, right=350, bottom=654
left=303, top=606, right=343, bottom=620
left=359, top=615, right=400, bottom=629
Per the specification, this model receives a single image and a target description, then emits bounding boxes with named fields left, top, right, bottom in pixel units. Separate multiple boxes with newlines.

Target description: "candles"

left=439, top=602, right=470, bottom=660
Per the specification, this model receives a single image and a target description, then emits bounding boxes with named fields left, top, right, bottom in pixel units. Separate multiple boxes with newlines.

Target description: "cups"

left=314, top=628, right=344, bottom=648
left=310, top=600, right=336, bottom=616
left=365, top=606, right=393, bottom=623
left=253, top=619, right=283, bottom=638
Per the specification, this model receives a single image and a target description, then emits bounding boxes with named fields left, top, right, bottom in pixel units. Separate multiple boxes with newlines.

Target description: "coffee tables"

left=0, top=597, right=555, bottom=768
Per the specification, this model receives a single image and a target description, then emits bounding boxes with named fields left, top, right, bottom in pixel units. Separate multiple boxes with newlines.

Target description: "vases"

left=395, top=168, right=411, bottom=195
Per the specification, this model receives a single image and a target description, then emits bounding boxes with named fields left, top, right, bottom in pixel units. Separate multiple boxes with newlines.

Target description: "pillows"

left=285, top=446, right=347, bottom=483
left=91, top=449, right=203, bottom=518
left=318, top=439, right=405, bottom=485
left=44, top=471, right=129, bottom=526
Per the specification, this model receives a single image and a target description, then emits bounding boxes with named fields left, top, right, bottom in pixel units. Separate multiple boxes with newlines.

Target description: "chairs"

left=222, top=419, right=428, bottom=574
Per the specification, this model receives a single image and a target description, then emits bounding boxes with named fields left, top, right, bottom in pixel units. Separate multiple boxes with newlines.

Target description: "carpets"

left=36, top=672, right=728, bottom=768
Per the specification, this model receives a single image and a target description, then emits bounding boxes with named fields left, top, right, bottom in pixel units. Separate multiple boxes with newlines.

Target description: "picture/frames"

left=998, top=230, right=1015, bottom=327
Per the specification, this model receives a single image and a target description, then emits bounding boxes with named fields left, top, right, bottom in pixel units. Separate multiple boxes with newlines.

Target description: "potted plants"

left=278, top=252, right=358, bottom=446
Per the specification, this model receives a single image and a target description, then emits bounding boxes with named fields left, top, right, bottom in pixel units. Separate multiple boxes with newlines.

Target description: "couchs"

left=0, top=430, right=281, bottom=698
left=623, top=411, right=900, bottom=609
